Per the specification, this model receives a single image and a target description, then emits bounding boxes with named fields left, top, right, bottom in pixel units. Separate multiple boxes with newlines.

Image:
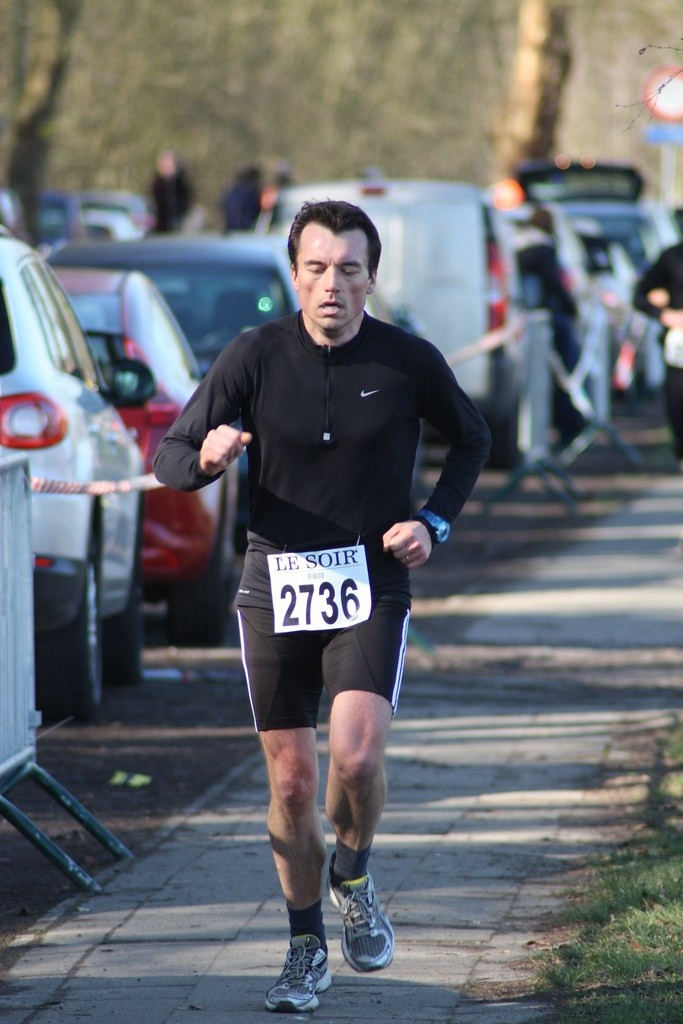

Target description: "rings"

left=405, top=554, right=412, bottom=563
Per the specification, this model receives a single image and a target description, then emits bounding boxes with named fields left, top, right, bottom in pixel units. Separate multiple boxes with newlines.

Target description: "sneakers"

left=264, top=934, right=332, bottom=1013
left=324, top=851, right=395, bottom=973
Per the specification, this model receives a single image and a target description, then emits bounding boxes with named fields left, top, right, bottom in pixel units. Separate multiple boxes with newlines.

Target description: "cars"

left=259, top=160, right=683, bottom=465
left=39, top=239, right=302, bottom=552
left=2, top=219, right=157, bottom=721
left=50, top=264, right=247, bottom=646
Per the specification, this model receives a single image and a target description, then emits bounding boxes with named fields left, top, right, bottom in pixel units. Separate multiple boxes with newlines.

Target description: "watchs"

left=417, top=509, right=451, bottom=544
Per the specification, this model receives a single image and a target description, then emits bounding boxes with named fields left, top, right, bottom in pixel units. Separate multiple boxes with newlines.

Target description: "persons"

left=635, top=209, right=683, bottom=466
left=154, top=202, right=492, bottom=1013
left=142, top=150, right=295, bottom=234
